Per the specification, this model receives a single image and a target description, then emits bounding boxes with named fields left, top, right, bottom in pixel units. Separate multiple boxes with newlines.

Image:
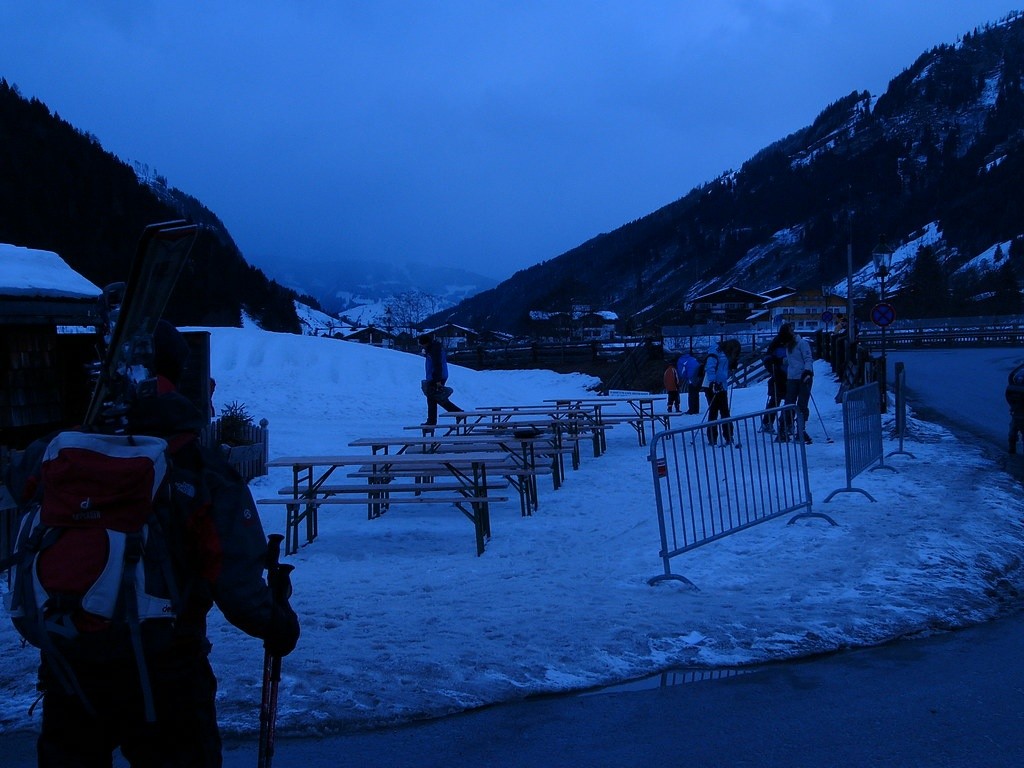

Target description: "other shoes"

left=777, top=431, right=791, bottom=443
left=762, top=423, right=775, bottom=433
left=796, top=431, right=811, bottom=443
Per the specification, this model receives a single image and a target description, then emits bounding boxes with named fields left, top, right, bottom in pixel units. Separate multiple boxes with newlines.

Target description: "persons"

left=11, top=318, right=300, bottom=768
left=761, top=323, right=814, bottom=442
left=702, top=339, right=742, bottom=449
left=834, top=312, right=849, bottom=333
left=1005, top=364, right=1024, bottom=453
left=663, top=353, right=704, bottom=414
left=418, top=334, right=464, bottom=426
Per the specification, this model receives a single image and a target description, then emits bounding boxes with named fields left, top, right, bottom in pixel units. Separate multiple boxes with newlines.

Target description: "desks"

left=543, top=397, right=667, bottom=442
left=476, top=403, right=617, bottom=457
left=347, top=434, right=556, bottom=516
left=440, top=408, right=594, bottom=470
left=403, top=418, right=580, bottom=488
left=262, top=451, right=513, bottom=551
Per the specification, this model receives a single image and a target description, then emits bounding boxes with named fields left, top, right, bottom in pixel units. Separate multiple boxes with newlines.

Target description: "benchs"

left=259, top=412, right=684, bottom=555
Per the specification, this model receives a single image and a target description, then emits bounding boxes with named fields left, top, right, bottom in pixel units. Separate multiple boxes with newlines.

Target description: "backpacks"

left=7, top=431, right=204, bottom=659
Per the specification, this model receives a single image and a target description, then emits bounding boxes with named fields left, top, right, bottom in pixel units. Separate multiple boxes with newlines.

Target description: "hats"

left=153, top=321, right=197, bottom=390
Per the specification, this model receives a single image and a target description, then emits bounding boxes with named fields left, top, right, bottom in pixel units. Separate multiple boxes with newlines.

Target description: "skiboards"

left=760, top=427, right=835, bottom=443
left=79, top=217, right=200, bottom=436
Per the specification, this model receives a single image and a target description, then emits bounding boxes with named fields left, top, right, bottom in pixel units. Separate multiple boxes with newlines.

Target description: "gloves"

left=803, top=373, right=811, bottom=384
left=773, top=357, right=783, bottom=368
left=766, top=358, right=774, bottom=365
left=262, top=608, right=299, bottom=658
left=709, top=382, right=721, bottom=394
